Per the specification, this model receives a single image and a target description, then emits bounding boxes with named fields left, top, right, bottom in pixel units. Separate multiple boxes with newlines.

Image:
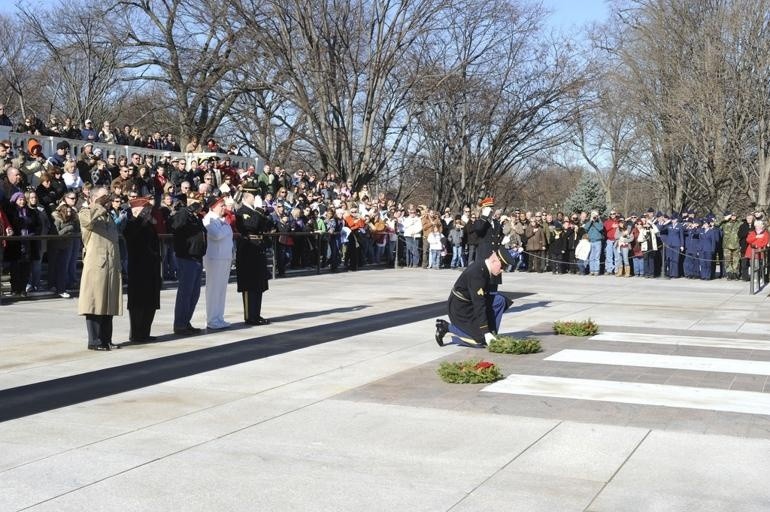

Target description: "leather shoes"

left=436, top=319, right=449, bottom=346
left=88, top=342, right=118, bottom=350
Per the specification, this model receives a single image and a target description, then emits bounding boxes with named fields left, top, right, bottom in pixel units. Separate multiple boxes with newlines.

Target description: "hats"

left=207, top=196, right=224, bottom=208
left=186, top=190, right=205, bottom=201
left=128, top=196, right=153, bottom=207
left=241, top=186, right=258, bottom=196
left=479, top=198, right=495, bottom=209
left=495, top=247, right=514, bottom=272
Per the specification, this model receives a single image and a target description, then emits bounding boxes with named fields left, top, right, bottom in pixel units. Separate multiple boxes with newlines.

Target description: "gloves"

left=484, top=332, right=496, bottom=345
left=95, top=193, right=112, bottom=207
left=254, top=194, right=262, bottom=209
left=226, top=214, right=235, bottom=224
left=481, top=207, right=492, bottom=217
